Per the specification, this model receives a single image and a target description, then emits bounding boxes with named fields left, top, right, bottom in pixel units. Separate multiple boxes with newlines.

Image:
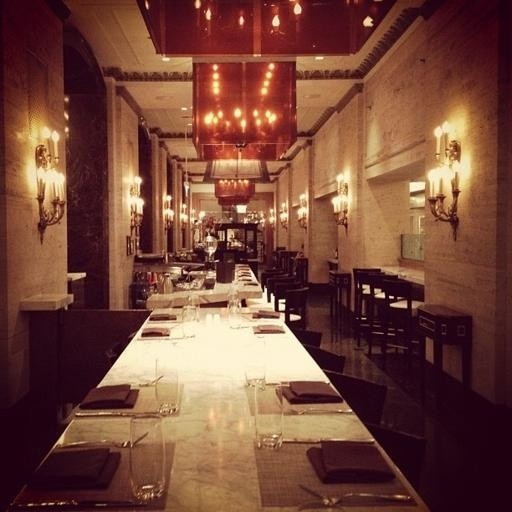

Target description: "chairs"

left=261, top=246, right=308, bottom=331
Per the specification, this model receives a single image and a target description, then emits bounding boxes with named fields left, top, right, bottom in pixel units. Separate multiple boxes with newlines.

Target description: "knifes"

left=280, top=437, right=376, bottom=445
left=0, top=499, right=153, bottom=510
left=75, top=409, right=160, bottom=419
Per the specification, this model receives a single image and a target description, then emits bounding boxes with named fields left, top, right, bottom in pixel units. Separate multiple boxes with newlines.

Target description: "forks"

left=131, top=370, right=164, bottom=388
left=283, top=404, right=353, bottom=415
left=53, top=429, right=151, bottom=450
left=295, top=480, right=413, bottom=506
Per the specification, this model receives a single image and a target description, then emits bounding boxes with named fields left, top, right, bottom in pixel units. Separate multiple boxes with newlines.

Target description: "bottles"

left=163, top=274, right=174, bottom=294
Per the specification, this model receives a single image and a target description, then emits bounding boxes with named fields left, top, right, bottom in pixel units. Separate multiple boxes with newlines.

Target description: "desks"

left=2, top=263, right=431, bottom=511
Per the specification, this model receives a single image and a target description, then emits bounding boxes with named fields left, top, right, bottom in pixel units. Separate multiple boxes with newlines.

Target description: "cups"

left=129, top=413, right=167, bottom=500
left=155, top=355, right=187, bottom=417
left=242, top=334, right=267, bottom=386
left=253, top=382, right=284, bottom=451
left=182, top=285, right=242, bottom=339
